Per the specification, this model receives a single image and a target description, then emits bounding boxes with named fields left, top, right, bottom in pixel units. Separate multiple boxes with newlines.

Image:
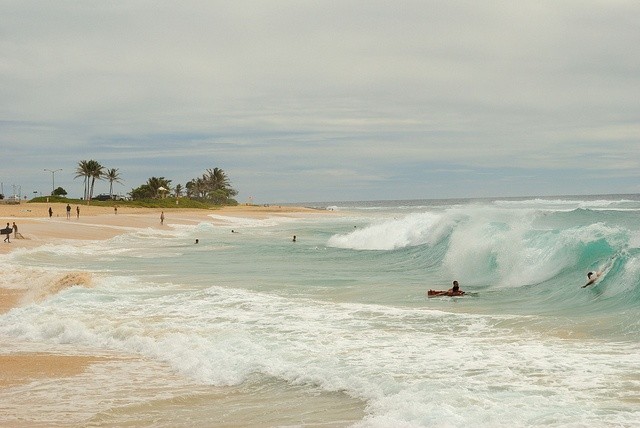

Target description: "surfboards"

left=427, top=289, right=448, bottom=296
left=0, top=228, right=13, bottom=235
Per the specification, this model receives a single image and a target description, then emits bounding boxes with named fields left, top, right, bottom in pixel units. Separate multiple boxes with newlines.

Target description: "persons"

left=11, top=222, right=17, bottom=239
left=452, top=281, right=459, bottom=292
left=231, top=230, right=239, bottom=233
left=160, top=211, right=164, bottom=223
left=194, top=239, right=198, bottom=244
left=76, top=206, right=80, bottom=218
left=66, top=204, right=71, bottom=217
left=292, top=236, right=296, bottom=242
left=3, top=222, right=11, bottom=243
left=114, top=207, right=118, bottom=214
left=49, top=207, right=53, bottom=217
left=580, top=269, right=603, bottom=289
